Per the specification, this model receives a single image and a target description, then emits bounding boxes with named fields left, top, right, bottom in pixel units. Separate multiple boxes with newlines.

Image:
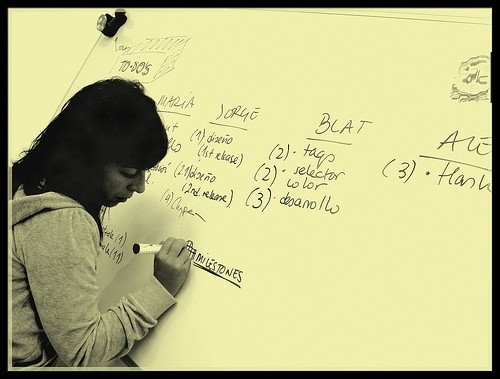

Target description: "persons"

left=13, top=76, right=190, bottom=366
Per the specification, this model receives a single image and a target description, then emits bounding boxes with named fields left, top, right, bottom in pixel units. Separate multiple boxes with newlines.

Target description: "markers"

left=132, top=244, right=163, bottom=256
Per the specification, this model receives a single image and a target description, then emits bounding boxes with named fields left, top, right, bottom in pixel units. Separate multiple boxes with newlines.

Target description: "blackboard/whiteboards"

left=10, top=9, right=492, bottom=370
